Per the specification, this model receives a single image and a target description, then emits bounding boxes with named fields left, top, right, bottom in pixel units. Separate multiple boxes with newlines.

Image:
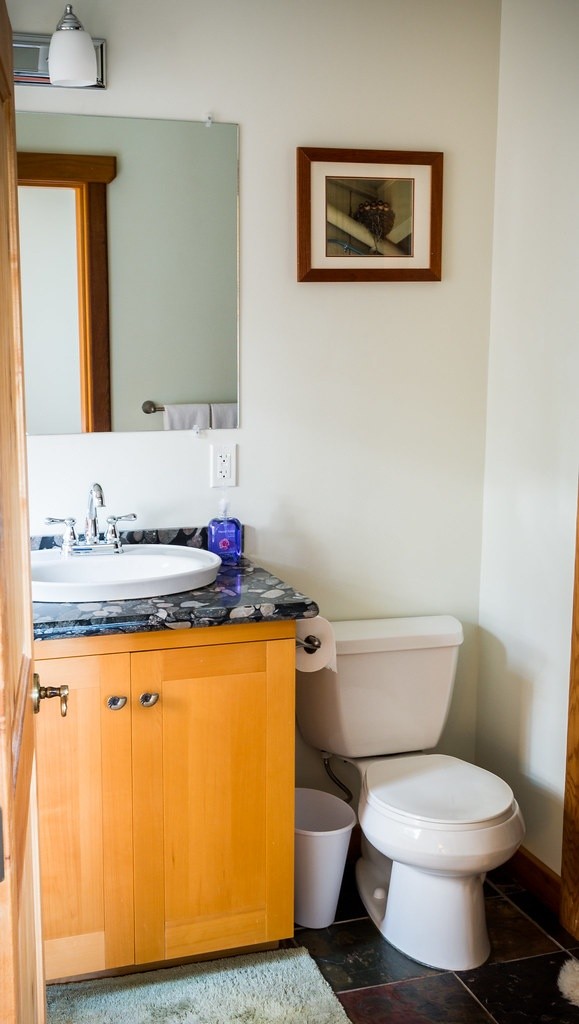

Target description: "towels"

left=163, top=404, right=211, bottom=431
left=212, top=403, right=238, bottom=429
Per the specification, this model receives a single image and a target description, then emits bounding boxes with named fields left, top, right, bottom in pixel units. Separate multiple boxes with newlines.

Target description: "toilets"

left=296, top=617, right=527, bottom=969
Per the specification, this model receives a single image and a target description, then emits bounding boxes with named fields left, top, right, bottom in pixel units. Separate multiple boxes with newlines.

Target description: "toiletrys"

left=207, top=500, right=244, bottom=565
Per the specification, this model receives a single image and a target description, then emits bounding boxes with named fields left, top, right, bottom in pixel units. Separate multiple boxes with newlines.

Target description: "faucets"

left=42, top=483, right=138, bottom=559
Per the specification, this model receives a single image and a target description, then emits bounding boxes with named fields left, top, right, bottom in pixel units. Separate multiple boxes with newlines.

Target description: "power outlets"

left=211, top=444, right=236, bottom=487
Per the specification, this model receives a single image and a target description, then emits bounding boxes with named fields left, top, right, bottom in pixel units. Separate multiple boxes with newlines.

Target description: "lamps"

left=48, top=3, right=97, bottom=87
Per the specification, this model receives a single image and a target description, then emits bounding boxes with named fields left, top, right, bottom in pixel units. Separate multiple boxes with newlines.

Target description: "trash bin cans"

left=295, top=787, right=358, bottom=930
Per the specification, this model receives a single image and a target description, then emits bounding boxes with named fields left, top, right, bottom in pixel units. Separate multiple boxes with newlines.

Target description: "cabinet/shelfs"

left=33, top=620, right=297, bottom=985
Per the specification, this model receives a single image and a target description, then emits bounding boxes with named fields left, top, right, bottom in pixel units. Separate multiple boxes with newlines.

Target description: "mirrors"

left=15, top=109, right=239, bottom=436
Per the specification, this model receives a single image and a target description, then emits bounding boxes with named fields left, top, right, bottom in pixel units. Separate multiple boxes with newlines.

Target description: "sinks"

left=32, top=543, right=223, bottom=602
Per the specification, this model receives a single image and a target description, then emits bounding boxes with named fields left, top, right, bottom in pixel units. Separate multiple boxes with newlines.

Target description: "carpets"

left=46, top=947, right=353, bottom=1024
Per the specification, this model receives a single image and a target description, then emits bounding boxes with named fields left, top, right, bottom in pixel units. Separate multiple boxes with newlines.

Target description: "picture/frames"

left=296, top=147, right=442, bottom=281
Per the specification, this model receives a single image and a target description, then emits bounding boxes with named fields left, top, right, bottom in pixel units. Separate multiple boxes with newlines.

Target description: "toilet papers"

left=296, top=613, right=338, bottom=672
